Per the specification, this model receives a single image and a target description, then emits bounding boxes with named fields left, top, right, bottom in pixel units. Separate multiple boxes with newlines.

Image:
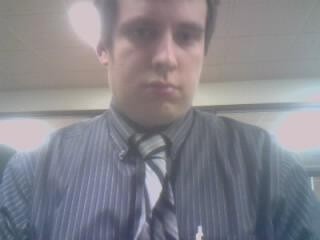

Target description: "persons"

left=1, top=0, right=320, bottom=240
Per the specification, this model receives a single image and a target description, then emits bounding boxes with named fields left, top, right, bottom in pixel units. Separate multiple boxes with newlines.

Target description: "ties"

left=134, top=130, right=180, bottom=240
